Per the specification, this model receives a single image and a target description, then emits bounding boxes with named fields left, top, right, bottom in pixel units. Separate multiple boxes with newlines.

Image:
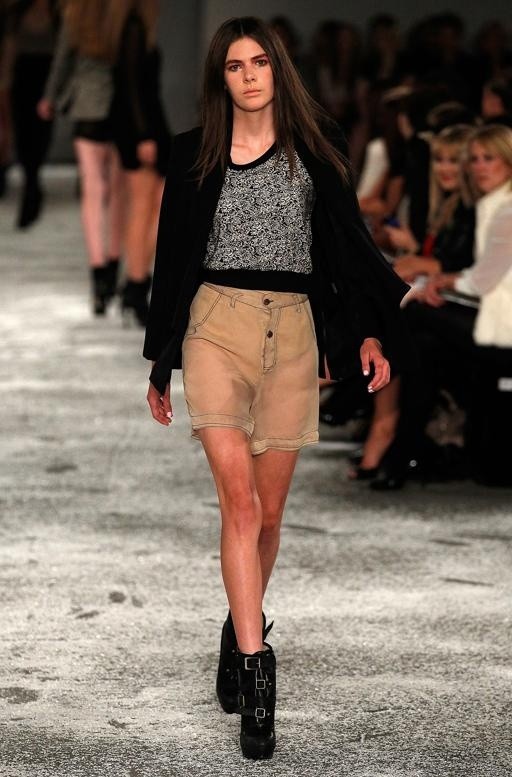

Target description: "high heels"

left=89, top=253, right=153, bottom=331
left=344, top=428, right=469, bottom=495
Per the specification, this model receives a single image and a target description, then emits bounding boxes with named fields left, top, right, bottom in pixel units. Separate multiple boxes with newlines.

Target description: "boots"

left=233, top=641, right=278, bottom=761
left=214, top=606, right=269, bottom=715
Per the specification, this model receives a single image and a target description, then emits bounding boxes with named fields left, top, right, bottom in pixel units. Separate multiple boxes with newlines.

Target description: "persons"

left=0, top=2, right=62, bottom=229
left=36, top=1, right=119, bottom=315
left=271, top=7, right=512, bottom=493
left=105, top=2, right=174, bottom=327
left=142, top=15, right=410, bottom=760
